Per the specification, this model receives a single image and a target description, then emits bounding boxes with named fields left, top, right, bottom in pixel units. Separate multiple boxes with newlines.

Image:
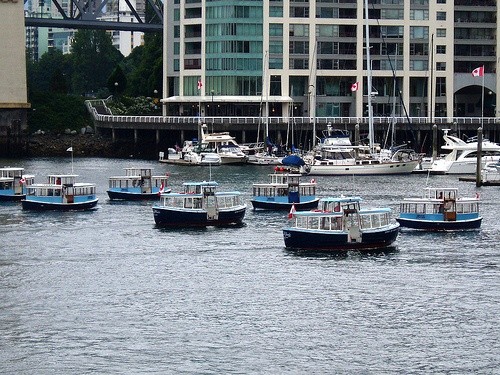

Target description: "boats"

left=394, top=168, right=483, bottom=233
left=250, top=167, right=320, bottom=211
left=106, top=168, right=172, bottom=201
left=158, top=0, right=499, bottom=175
left=151, top=162, right=247, bottom=228
left=20, top=147, right=100, bottom=211
left=482, top=156, right=500, bottom=186
left=0, top=165, right=36, bottom=201
left=282, top=172, right=401, bottom=252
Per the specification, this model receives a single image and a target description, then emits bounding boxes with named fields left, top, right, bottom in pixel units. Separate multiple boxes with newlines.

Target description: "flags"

left=198, top=80, right=202, bottom=89
left=19, top=178, right=25, bottom=185
left=351, top=82, right=359, bottom=91
left=472, top=67, right=483, bottom=77
left=289, top=204, right=296, bottom=219
left=159, top=182, right=164, bottom=196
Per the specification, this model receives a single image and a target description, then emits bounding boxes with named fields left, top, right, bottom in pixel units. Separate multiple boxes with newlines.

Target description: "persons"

left=56, top=178, right=61, bottom=184
left=439, top=192, right=445, bottom=209
